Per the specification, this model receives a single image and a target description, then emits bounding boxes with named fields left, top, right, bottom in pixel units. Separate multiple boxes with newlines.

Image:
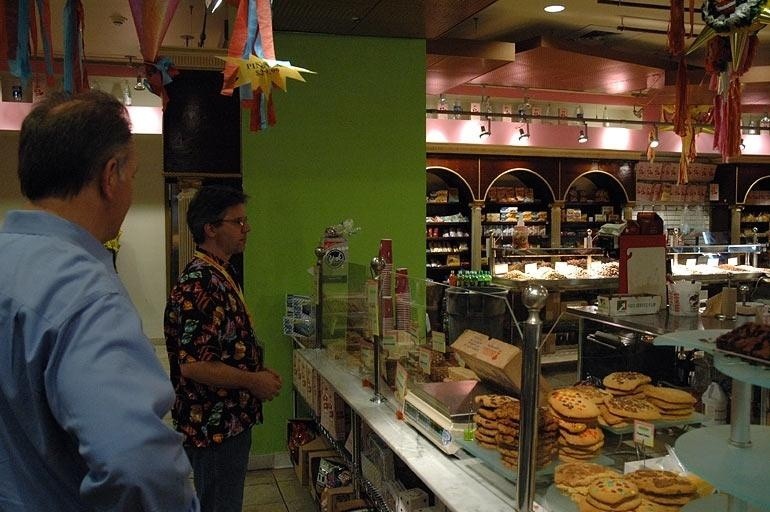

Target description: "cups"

left=375, top=236, right=411, bottom=333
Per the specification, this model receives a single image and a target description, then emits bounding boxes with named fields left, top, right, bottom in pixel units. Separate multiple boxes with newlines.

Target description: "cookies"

left=471, top=371, right=703, bottom=512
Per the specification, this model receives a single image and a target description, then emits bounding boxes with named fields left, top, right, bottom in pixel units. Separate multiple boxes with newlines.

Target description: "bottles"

left=449, top=269, right=493, bottom=288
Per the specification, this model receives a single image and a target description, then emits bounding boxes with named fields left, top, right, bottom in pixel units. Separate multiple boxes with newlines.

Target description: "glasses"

left=221, top=216, right=247, bottom=225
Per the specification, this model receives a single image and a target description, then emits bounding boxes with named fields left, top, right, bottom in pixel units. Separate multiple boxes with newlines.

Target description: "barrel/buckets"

left=701, top=380, right=729, bottom=424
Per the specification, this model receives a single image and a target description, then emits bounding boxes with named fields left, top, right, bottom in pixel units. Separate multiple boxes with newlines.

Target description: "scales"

left=401, top=379, right=495, bottom=455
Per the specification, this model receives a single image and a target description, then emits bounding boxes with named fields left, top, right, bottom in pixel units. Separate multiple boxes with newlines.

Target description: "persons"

left=1, top=88, right=201, bottom=512
left=164, top=186, right=282, bottom=512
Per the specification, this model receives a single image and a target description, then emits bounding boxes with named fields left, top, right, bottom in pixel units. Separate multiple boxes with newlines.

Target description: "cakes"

left=716, top=321, right=770, bottom=367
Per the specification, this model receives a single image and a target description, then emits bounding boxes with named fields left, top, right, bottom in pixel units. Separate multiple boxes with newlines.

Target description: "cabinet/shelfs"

left=419, top=160, right=633, bottom=276
left=289, top=323, right=770, bottom=512
left=726, top=191, right=769, bottom=271
